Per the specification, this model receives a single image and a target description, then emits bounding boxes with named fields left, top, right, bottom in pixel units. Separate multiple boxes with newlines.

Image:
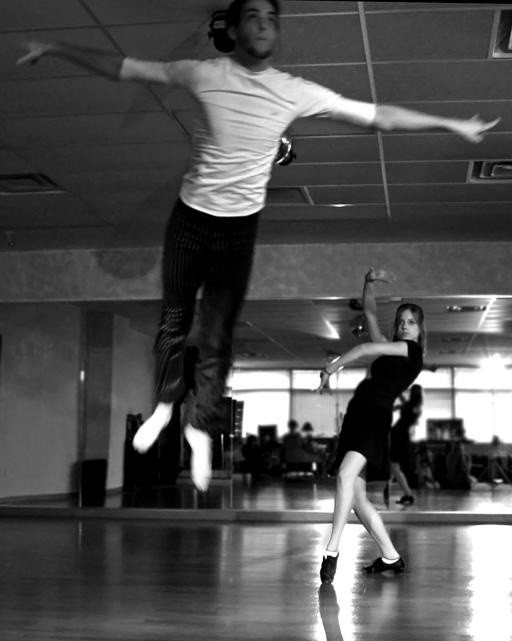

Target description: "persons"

left=239, top=382, right=423, bottom=505
left=13, top=0, right=504, bottom=492
left=309, top=264, right=426, bottom=585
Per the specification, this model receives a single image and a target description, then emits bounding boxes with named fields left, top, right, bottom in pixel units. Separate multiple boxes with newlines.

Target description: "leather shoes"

left=320, top=552, right=339, bottom=585
left=383, top=482, right=391, bottom=504
left=363, top=557, right=405, bottom=575
left=396, top=495, right=415, bottom=504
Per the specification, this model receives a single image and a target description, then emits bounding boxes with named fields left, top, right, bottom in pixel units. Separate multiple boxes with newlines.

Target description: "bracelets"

left=365, top=273, right=374, bottom=283
left=323, top=366, right=331, bottom=376
